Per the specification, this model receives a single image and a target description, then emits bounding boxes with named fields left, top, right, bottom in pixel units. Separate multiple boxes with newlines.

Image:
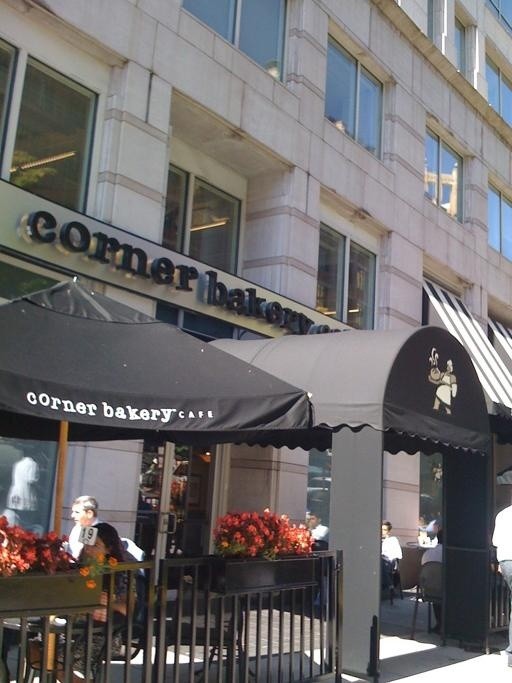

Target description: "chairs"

left=410, top=560, right=443, bottom=640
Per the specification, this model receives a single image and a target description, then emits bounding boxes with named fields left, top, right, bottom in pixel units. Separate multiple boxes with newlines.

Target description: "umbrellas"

left=0, top=276, right=311, bottom=683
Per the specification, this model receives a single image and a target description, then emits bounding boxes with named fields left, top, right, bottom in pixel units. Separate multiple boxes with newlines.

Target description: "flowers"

left=212, top=507, right=315, bottom=560
left=0, top=515, right=119, bottom=588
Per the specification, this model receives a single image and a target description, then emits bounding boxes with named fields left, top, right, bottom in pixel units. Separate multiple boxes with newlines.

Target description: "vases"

left=198, top=554, right=317, bottom=596
left=0, top=568, right=105, bottom=616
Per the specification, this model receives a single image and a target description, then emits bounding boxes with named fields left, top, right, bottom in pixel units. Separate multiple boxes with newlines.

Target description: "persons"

left=307, top=507, right=512, bottom=667
left=4, top=444, right=42, bottom=528
left=28, top=494, right=144, bottom=683
